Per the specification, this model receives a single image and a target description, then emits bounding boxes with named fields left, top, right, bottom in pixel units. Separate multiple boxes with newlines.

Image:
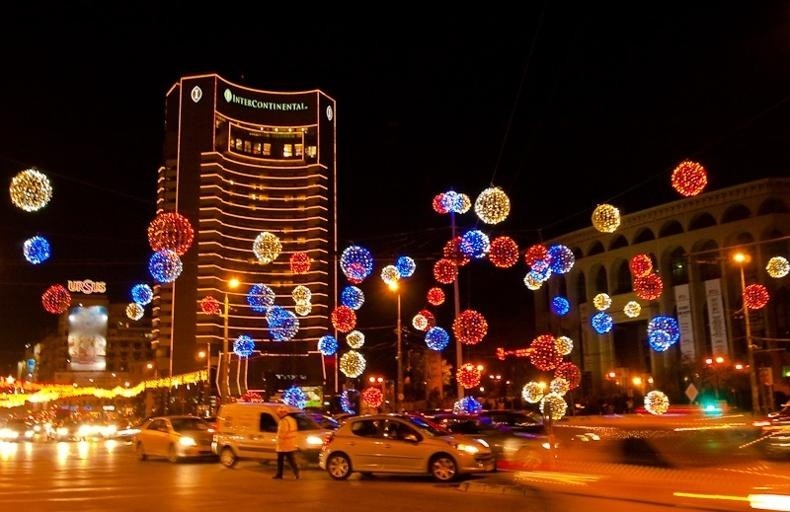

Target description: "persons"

left=271, top=407, right=302, bottom=480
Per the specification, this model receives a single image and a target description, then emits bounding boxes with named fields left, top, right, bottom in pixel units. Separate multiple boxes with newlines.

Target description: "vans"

left=210, top=402, right=336, bottom=469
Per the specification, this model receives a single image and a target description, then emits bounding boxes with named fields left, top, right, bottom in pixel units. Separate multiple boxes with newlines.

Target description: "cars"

left=0, top=419, right=143, bottom=442
left=132, top=415, right=216, bottom=463
left=317, top=410, right=563, bottom=482
left=756, top=401, right=790, bottom=460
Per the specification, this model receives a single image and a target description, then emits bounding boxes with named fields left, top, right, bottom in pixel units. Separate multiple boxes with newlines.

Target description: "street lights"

left=387, top=278, right=404, bottom=414
left=220, top=278, right=240, bottom=405
left=731, top=251, right=762, bottom=419
